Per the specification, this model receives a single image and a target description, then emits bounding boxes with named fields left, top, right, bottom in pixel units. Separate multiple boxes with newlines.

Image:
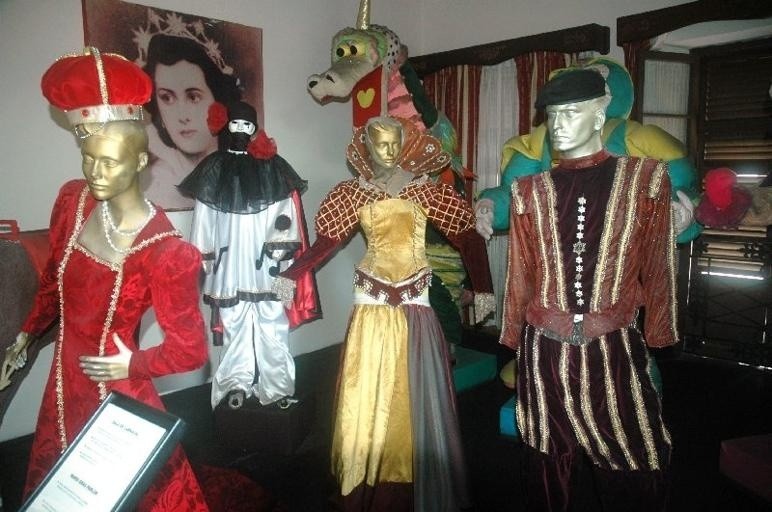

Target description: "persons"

left=258, top=114, right=498, bottom=508
left=183, top=101, right=322, bottom=413
left=496, top=70, right=684, bottom=504
left=474, top=53, right=717, bottom=389
left=113, top=8, right=245, bottom=208
left=10, top=43, right=211, bottom=510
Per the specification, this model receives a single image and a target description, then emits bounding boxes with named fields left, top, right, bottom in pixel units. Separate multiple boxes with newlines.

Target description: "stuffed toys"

left=304, top=1, right=480, bottom=354
